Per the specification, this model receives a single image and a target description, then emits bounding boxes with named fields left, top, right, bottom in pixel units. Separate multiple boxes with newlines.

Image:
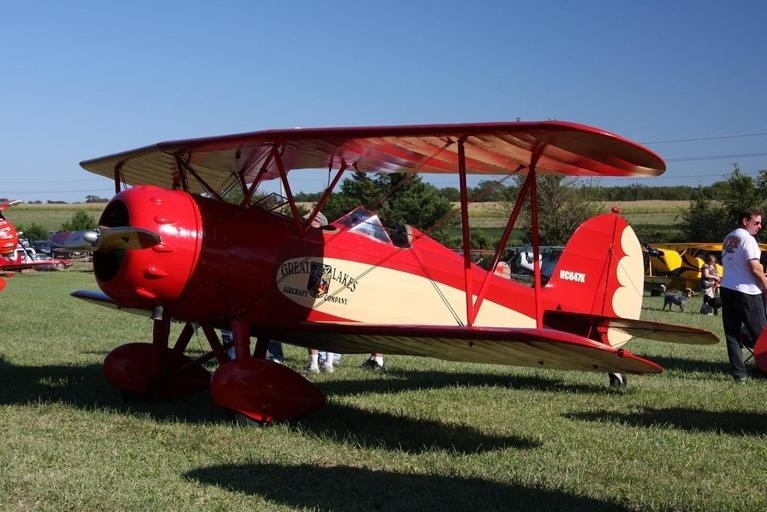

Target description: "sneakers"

left=302, top=363, right=334, bottom=373
left=362, top=358, right=381, bottom=368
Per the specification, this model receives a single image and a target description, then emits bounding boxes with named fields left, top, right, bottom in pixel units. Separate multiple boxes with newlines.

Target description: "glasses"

left=755, top=222, right=759, bottom=225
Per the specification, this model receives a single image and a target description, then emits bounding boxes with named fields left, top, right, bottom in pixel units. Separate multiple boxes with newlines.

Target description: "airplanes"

left=47, top=121, right=720, bottom=427
left=506, top=246, right=565, bottom=278
left=641, top=243, right=767, bottom=298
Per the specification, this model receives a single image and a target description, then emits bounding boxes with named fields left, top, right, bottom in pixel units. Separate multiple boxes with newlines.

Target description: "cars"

left=8, top=240, right=74, bottom=269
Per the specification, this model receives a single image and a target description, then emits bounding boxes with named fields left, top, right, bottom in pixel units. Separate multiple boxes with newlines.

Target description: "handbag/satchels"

left=701, top=278, right=713, bottom=289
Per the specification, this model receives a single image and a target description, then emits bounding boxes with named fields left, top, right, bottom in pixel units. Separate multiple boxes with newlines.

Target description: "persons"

left=220, top=329, right=385, bottom=378
left=707, top=254, right=719, bottom=316
left=700, top=256, right=721, bottom=316
left=719, top=207, right=766, bottom=386
